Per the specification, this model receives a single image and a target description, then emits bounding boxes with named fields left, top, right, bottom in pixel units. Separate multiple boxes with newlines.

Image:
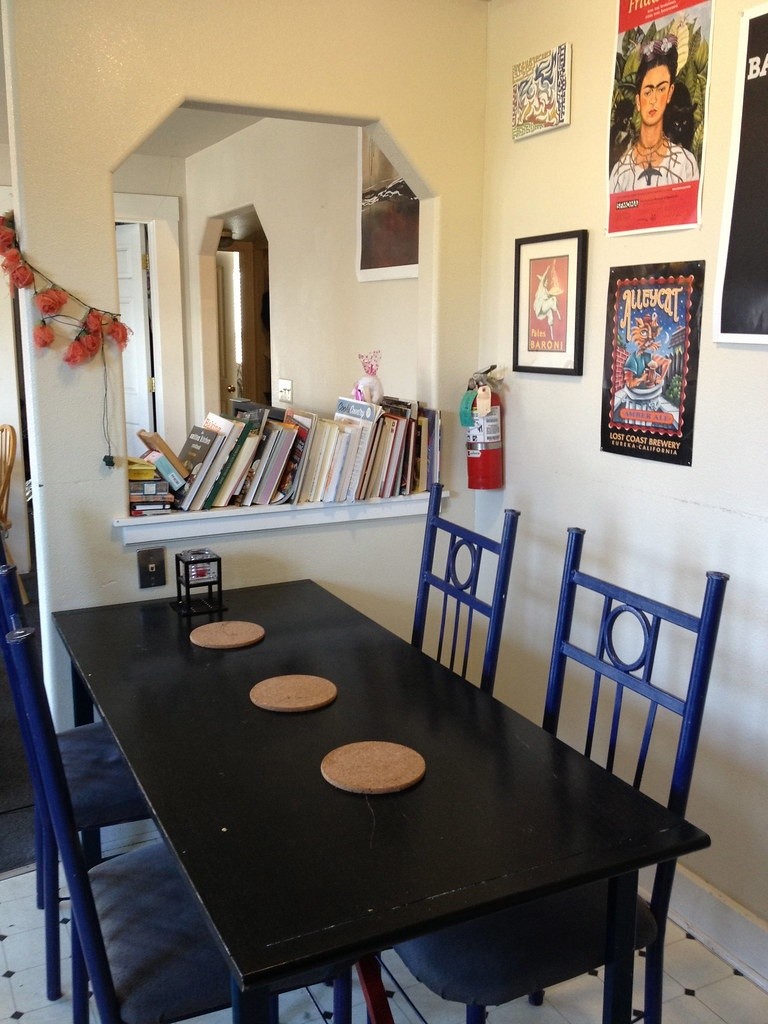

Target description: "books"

left=124, top=393, right=438, bottom=517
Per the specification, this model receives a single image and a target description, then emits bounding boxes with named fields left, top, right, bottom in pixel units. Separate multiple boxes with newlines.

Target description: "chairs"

left=7, top=628, right=353, bottom=1024
left=374, top=528, right=731, bottom=1024
left=0, top=563, right=153, bottom=1000
left=412, top=483, right=520, bottom=696
left=1, top=424, right=30, bottom=606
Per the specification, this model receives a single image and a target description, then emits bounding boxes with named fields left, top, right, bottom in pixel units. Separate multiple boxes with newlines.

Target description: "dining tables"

left=51, top=579, right=712, bottom=1024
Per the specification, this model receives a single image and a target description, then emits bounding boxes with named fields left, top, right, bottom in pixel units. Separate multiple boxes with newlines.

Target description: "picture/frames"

left=358, top=125, right=419, bottom=282
left=512, top=230, right=589, bottom=376
left=711, top=9, right=768, bottom=344
left=607, top=0, right=716, bottom=238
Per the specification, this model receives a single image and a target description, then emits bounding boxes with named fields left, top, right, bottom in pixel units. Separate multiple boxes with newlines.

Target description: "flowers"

left=0, top=210, right=128, bottom=364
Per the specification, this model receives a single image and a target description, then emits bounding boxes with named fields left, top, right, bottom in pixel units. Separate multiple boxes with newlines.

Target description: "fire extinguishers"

left=459, top=364, right=504, bottom=490
